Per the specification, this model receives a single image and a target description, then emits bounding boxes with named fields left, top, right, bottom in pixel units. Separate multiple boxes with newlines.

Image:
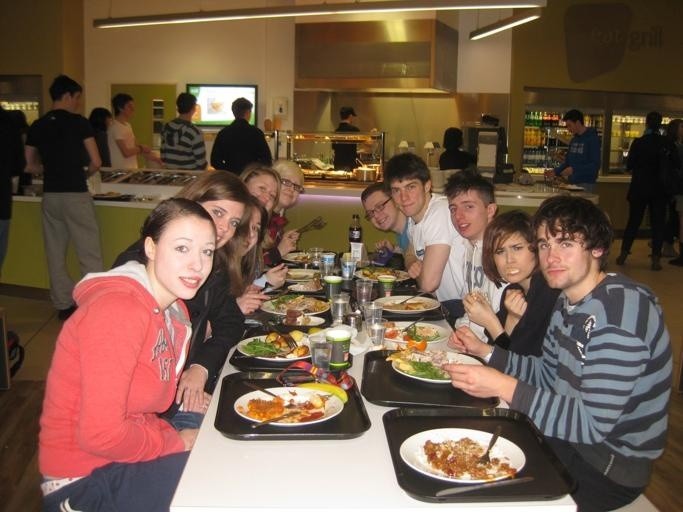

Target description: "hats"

left=340, top=106, right=357, bottom=117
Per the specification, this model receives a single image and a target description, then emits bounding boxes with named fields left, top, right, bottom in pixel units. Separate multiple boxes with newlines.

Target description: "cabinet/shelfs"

left=270, top=128, right=387, bottom=187
left=609, top=111, right=682, bottom=182
left=522, top=103, right=608, bottom=183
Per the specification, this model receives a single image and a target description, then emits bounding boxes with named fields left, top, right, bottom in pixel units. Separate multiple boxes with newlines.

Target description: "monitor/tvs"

left=190, top=83, right=259, bottom=129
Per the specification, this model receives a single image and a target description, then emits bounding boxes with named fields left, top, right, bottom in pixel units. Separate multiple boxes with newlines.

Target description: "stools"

left=615, top=493, right=659, bottom=512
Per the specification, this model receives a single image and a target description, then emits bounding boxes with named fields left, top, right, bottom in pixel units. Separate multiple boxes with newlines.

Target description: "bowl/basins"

left=352, top=162, right=381, bottom=181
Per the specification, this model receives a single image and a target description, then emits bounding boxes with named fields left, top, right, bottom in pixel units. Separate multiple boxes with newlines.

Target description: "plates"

left=236, top=335, right=311, bottom=363
left=256, top=250, right=331, bottom=327
left=371, top=290, right=486, bottom=388
left=232, top=387, right=344, bottom=426
left=354, top=264, right=410, bottom=283
left=396, top=426, right=527, bottom=485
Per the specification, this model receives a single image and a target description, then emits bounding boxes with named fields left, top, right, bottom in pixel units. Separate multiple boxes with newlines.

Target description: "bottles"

left=348, top=213, right=362, bottom=253
left=522, top=110, right=602, bottom=165
left=612, top=117, right=671, bottom=138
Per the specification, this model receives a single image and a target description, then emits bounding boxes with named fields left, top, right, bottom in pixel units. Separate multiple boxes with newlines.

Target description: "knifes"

left=251, top=409, right=301, bottom=431
left=434, top=476, right=534, bottom=497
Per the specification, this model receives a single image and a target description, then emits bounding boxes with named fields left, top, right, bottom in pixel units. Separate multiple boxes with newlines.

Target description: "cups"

left=307, top=245, right=386, bottom=375
left=376, top=273, right=396, bottom=298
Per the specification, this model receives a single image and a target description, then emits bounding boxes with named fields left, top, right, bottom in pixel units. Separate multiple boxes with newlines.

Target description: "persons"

left=160, top=90, right=207, bottom=169
left=459, top=207, right=569, bottom=362
left=89, top=93, right=162, bottom=169
left=23, top=72, right=101, bottom=318
left=0, top=108, right=27, bottom=260
left=556, top=110, right=682, bottom=272
left=210, top=98, right=272, bottom=167
left=331, top=106, right=373, bottom=173
left=439, top=115, right=508, bottom=169
left=362, top=152, right=506, bottom=345
left=35, top=194, right=219, bottom=511
left=208, top=158, right=306, bottom=337
left=438, top=192, right=675, bottom=510
left=0, top=319, right=27, bottom=389
left=109, top=169, right=250, bottom=414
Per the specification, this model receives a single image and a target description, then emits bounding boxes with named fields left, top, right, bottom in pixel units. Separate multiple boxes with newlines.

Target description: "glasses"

left=364, top=196, right=392, bottom=220
left=281, top=178, right=304, bottom=193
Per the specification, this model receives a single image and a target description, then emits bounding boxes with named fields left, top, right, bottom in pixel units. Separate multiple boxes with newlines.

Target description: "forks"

left=262, top=321, right=297, bottom=348
left=475, top=424, right=503, bottom=465
left=242, top=379, right=297, bottom=409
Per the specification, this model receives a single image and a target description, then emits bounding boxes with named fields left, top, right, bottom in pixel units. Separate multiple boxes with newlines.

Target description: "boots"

left=616, top=239, right=683, bottom=270
